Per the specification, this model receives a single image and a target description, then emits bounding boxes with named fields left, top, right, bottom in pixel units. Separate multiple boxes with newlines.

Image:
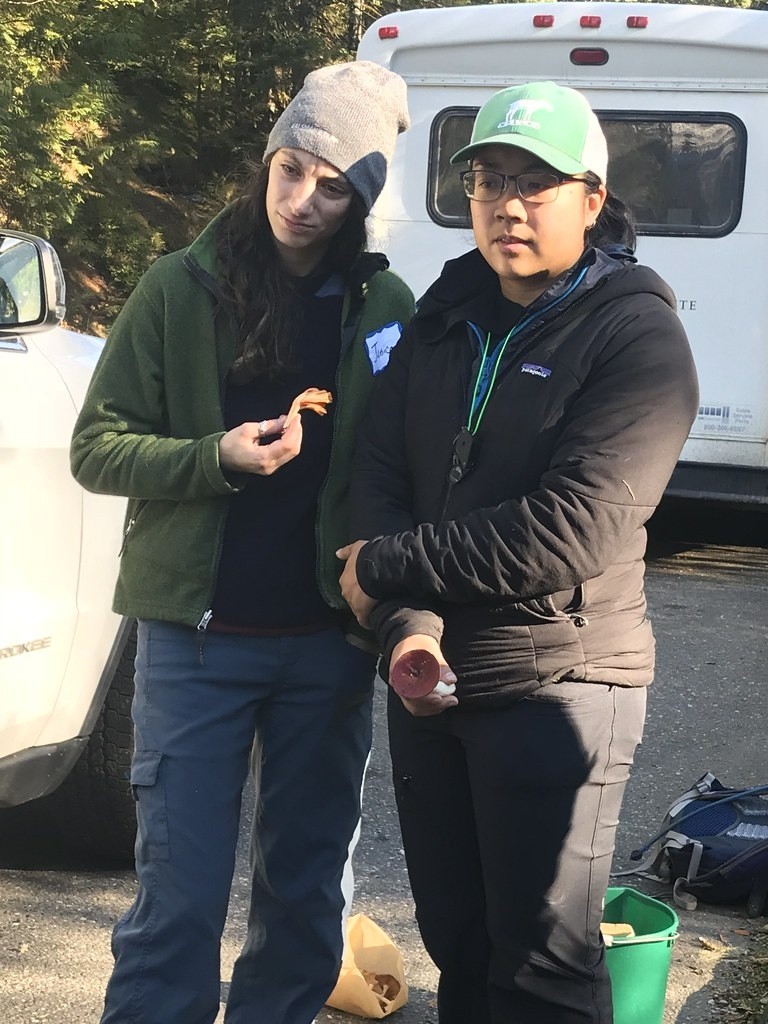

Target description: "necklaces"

left=447, top=327, right=514, bottom=485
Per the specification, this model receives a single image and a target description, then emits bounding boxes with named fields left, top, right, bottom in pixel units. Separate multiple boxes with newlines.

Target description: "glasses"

left=460, top=169, right=598, bottom=203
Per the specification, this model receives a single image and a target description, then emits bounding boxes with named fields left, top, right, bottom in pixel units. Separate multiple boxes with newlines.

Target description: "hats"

left=262, top=60, right=410, bottom=216
left=450, top=81, right=609, bottom=187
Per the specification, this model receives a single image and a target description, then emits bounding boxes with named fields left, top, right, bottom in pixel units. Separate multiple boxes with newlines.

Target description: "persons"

left=69, top=64, right=416, bottom=1024
left=336, top=82, right=699, bottom=1024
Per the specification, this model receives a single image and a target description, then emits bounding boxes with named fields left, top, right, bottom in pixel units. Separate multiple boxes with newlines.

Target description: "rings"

left=260, top=420, right=267, bottom=438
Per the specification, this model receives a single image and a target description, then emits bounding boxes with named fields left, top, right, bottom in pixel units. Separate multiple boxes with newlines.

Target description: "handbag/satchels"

left=325, top=913, right=408, bottom=1018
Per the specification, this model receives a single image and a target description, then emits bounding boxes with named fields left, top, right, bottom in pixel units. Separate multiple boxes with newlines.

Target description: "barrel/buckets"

left=601, top=887, right=679, bottom=1023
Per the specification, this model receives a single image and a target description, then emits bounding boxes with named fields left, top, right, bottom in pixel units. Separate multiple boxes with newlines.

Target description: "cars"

left=0, top=229, right=140, bottom=875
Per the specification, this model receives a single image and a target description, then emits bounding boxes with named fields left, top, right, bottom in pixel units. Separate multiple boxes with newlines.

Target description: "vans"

left=354, top=1, right=767, bottom=511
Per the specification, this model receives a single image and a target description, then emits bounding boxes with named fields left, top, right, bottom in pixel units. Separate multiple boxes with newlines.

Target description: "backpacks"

left=610, top=772, right=768, bottom=918
